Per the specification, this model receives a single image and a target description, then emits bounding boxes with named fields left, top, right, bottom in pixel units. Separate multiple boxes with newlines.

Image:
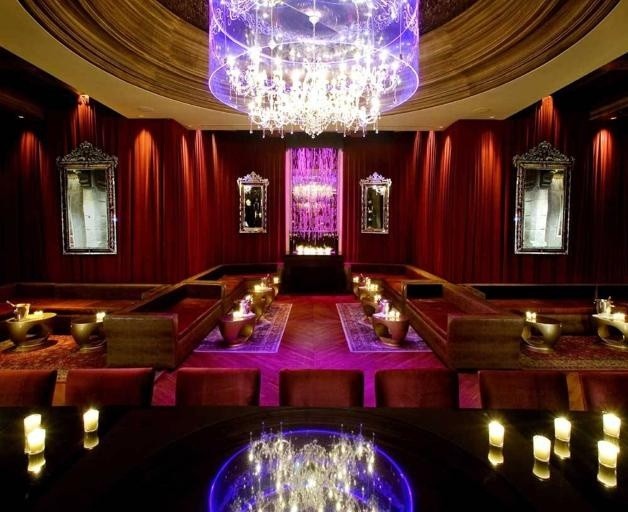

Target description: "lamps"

left=289, top=147, right=339, bottom=243
left=205, top=1, right=421, bottom=143
left=206, top=418, right=415, bottom=512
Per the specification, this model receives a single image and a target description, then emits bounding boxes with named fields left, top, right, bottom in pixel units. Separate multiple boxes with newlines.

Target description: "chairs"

left=476, top=369, right=572, bottom=410
left=374, top=368, right=463, bottom=410
left=579, top=370, right=628, bottom=411
left=0, top=366, right=57, bottom=413
left=277, top=367, right=364, bottom=413
left=64, top=368, right=157, bottom=412
left=176, top=367, right=261, bottom=411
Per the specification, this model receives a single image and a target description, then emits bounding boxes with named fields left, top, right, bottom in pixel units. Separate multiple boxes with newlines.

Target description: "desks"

left=520, top=316, right=563, bottom=356
left=352, top=274, right=411, bottom=347
left=0, top=402, right=627, bottom=512
left=218, top=276, right=283, bottom=345
left=590, top=311, right=627, bottom=352
left=71, top=317, right=107, bottom=350
left=3, top=311, right=59, bottom=353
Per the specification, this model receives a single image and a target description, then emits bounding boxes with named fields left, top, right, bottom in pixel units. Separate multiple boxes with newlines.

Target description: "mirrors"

left=236, top=170, right=269, bottom=234
left=358, top=171, right=393, bottom=236
left=56, top=141, right=120, bottom=256
left=285, top=146, right=344, bottom=255
left=511, top=141, right=576, bottom=256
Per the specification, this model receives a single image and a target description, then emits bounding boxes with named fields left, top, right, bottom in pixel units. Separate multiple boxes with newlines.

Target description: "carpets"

left=334, top=301, right=435, bottom=354
left=516, top=332, right=628, bottom=370
left=0, top=335, right=167, bottom=387
left=192, top=301, right=294, bottom=353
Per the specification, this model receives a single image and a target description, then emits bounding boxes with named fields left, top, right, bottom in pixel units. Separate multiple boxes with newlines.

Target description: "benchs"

left=1, top=279, right=172, bottom=330
left=465, top=281, right=627, bottom=335
left=102, top=282, right=231, bottom=367
left=402, top=280, right=523, bottom=369
left=187, top=262, right=282, bottom=295
left=342, top=261, right=444, bottom=310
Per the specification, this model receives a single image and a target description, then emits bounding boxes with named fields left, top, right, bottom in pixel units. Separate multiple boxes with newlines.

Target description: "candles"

left=27, top=427, right=47, bottom=455
left=485, top=412, right=623, bottom=472
left=22, top=413, right=42, bottom=436
left=82, top=407, right=99, bottom=434
